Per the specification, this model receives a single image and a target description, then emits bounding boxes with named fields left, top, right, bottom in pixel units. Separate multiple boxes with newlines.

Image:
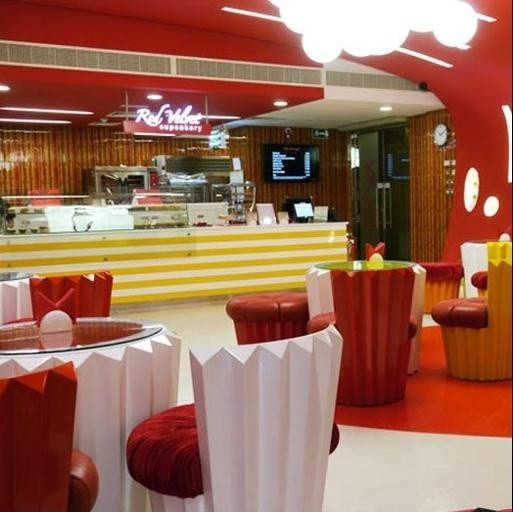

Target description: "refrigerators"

left=146, top=155, right=246, bottom=204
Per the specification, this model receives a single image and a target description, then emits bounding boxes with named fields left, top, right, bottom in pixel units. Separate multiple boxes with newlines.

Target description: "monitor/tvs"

left=264, top=143, right=320, bottom=182
left=292, top=202, right=313, bottom=222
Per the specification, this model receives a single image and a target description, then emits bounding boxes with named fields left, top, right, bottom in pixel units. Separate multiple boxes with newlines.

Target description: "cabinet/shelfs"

left=0, top=185, right=191, bottom=238
left=85, top=164, right=149, bottom=205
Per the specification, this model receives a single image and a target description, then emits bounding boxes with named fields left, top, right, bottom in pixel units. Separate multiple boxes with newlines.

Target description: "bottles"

left=3, top=209, right=48, bottom=234
left=228, top=208, right=244, bottom=224
left=72, top=207, right=92, bottom=232
left=194, top=214, right=205, bottom=226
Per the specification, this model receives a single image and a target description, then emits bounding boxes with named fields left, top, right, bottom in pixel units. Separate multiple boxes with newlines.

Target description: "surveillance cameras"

left=285, top=128, right=292, bottom=134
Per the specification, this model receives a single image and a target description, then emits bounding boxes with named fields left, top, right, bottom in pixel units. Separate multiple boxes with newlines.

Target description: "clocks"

left=431, top=121, right=451, bottom=149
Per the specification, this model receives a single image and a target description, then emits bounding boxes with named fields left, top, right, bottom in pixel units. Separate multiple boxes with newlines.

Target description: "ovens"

left=85, top=164, right=159, bottom=206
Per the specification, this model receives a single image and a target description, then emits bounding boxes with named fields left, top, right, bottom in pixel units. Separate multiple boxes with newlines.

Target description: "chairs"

left=1, top=272, right=344, bottom=511
left=305, top=241, right=513, bottom=406
